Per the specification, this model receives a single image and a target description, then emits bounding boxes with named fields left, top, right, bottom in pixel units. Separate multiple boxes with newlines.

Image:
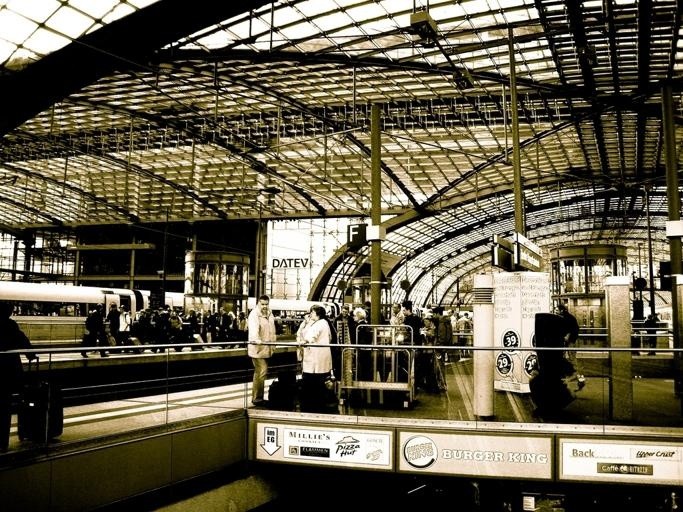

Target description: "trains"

left=0, top=281, right=473, bottom=349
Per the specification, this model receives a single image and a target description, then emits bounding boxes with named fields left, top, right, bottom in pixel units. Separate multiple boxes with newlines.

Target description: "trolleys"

left=338, top=323, right=415, bottom=410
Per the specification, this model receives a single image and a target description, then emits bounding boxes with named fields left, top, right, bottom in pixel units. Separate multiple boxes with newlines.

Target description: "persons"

left=644, top=315, right=661, bottom=356
left=79, top=302, right=304, bottom=359
left=0, top=298, right=35, bottom=454
left=299, top=306, right=332, bottom=414
left=293, top=299, right=474, bottom=401
left=553, top=303, right=580, bottom=382
left=246, top=294, right=277, bottom=405
